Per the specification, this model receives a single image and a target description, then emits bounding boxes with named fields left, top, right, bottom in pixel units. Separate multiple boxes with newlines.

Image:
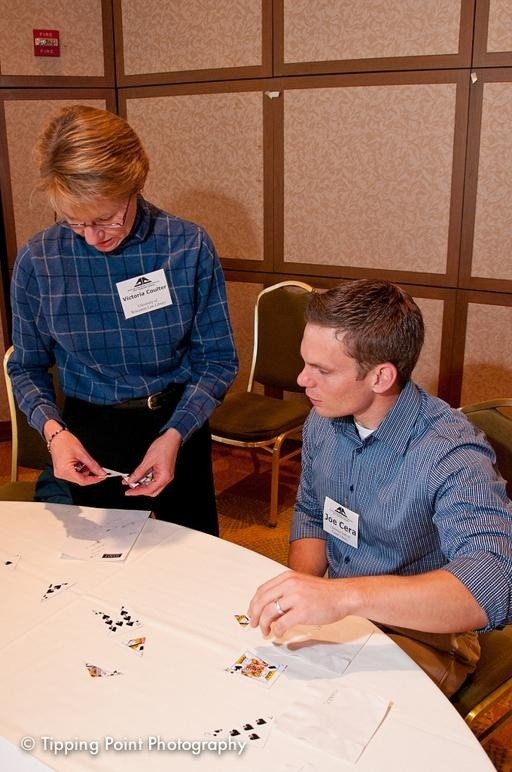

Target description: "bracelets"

left=43, top=424, right=66, bottom=456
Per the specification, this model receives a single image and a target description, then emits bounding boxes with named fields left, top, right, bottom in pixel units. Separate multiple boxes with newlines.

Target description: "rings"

left=273, top=601, right=284, bottom=617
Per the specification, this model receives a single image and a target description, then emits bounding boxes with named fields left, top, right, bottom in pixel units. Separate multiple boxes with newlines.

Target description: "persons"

left=249, top=281, right=511, bottom=703
left=6, top=106, right=240, bottom=539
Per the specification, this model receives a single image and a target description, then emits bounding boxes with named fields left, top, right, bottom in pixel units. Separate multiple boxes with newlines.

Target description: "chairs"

left=3, top=346, right=64, bottom=480
left=450, top=398, right=512, bottom=772
left=209, top=279, right=311, bottom=528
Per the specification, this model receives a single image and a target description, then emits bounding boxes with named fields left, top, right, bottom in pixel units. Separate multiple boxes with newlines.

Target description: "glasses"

left=53, top=189, right=133, bottom=231
